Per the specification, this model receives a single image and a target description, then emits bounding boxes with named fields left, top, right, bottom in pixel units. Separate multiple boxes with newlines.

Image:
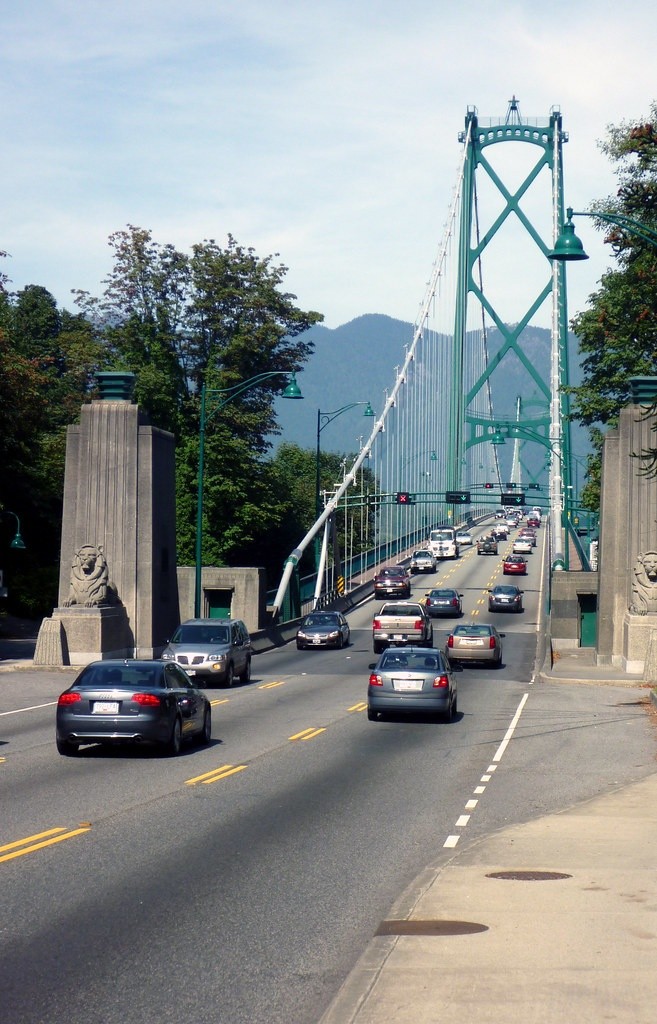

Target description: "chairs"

left=518, top=559, right=521, bottom=562
left=509, top=558, right=512, bottom=562
left=138, top=673, right=169, bottom=687
left=100, top=669, right=123, bottom=685
left=424, top=657, right=436, bottom=668
left=496, top=588, right=502, bottom=593
left=433, top=592, right=439, bottom=596
left=386, top=656, right=408, bottom=668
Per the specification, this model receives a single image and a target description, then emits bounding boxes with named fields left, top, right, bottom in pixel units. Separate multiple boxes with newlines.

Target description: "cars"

left=443, top=624, right=506, bottom=670
left=295, top=610, right=351, bottom=650
left=159, top=618, right=253, bottom=688
left=425, top=589, right=464, bottom=617
left=56, top=658, right=213, bottom=757
left=366, top=647, right=465, bottom=724
left=409, top=507, right=544, bottom=613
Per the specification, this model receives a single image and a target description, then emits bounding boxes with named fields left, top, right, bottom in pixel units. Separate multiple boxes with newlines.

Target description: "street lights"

left=315, top=400, right=376, bottom=570
left=396, top=447, right=438, bottom=536
left=490, top=422, right=570, bottom=571
left=193, top=367, right=305, bottom=618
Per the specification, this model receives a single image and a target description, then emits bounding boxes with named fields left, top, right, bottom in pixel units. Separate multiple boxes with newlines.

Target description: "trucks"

left=427, top=526, right=462, bottom=559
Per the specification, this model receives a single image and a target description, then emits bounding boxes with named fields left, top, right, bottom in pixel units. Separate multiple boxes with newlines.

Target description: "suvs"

left=375, top=565, right=412, bottom=598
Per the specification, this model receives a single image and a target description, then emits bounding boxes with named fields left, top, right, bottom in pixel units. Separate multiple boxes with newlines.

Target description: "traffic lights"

left=485, top=483, right=493, bottom=488
left=501, top=494, right=526, bottom=506
left=529, top=483, right=540, bottom=489
left=445, top=491, right=471, bottom=503
left=506, top=483, right=516, bottom=488
left=398, top=492, right=409, bottom=504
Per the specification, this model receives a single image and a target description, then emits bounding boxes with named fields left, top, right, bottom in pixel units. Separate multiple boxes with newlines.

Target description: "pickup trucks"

left=371, top=600, right=434, bottom=653
